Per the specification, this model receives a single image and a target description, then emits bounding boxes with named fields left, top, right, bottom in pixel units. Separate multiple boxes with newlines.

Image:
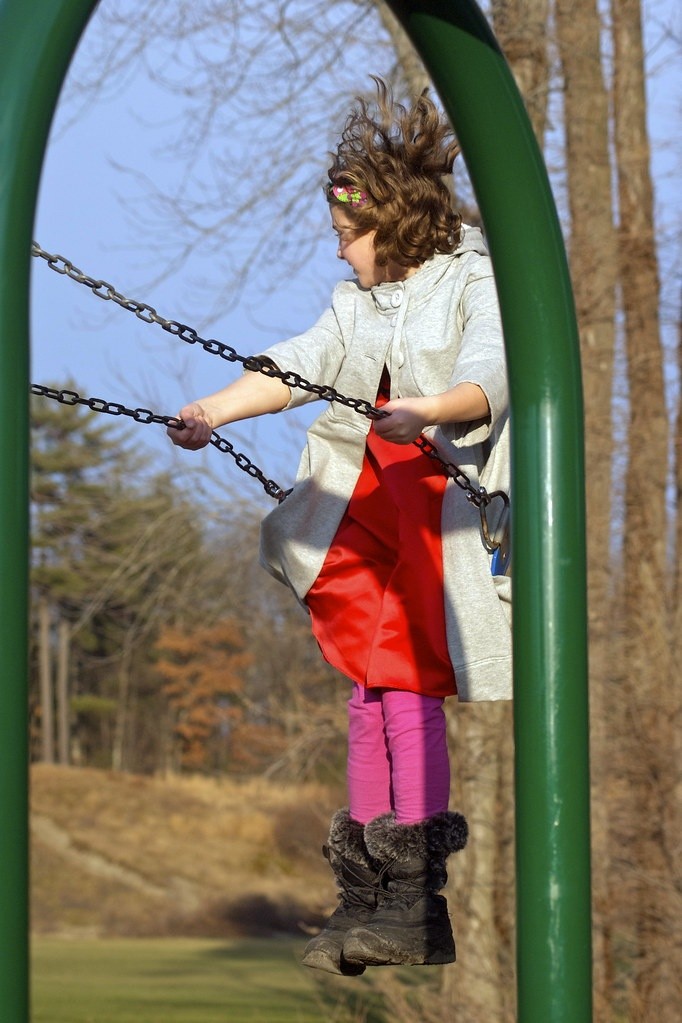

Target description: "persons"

left=166, top=76, right=514, bottom=977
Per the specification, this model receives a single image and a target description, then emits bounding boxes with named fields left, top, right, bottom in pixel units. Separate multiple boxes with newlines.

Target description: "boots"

left=343, top=808, right=469, bottom=965
left=301, top=807, right=382, bottom=977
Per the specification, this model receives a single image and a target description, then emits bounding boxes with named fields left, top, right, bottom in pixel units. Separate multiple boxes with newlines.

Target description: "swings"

left=29, top=241, right=513, bottom=581
